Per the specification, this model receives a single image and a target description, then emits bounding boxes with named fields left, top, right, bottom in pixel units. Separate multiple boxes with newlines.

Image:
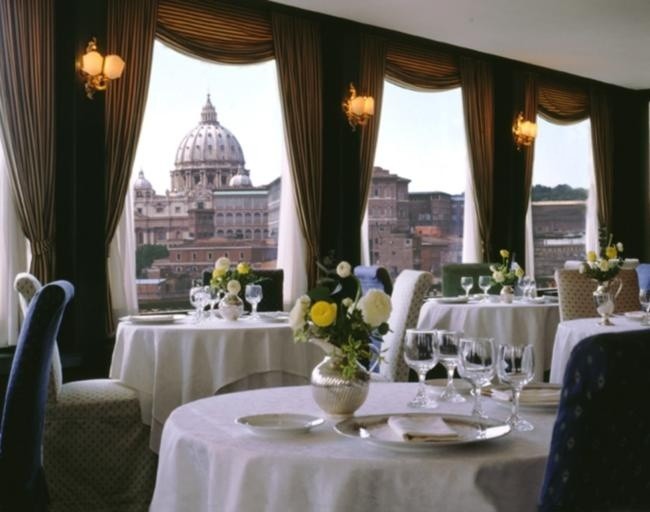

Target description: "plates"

left=482, top=379, right=562, bottom=410
left=624, top=309, right=645, bottom=319
left=435, top=296, right=470, bottom=304
left=118, top=314, right=186, bottom=324
left=331, top=412, right=510, bottom=453
left=234, top=413, right=323, bottom=436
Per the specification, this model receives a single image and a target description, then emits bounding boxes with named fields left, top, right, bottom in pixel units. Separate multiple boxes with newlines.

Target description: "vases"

left=592, top=278, right=626, bottom=326
left=500, top=286, right=515, bottom=303
left=309, top=347, right=378, bottom=422
left=219, top=292, right=243, bottom=320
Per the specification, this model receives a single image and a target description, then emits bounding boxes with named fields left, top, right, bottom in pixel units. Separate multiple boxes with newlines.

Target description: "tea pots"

left=306, top=334, right=382, bottom=421
left=589, top=274, right=624, bottom=328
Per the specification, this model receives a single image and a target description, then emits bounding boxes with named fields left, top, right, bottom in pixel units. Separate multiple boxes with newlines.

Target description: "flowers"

left=289, top=257, right=392, bottom=380
left=575, top=240, right=628, bottom=276
left=489, top=250, right=524, bottom=283
left=210, top=257, right=268, bottom=292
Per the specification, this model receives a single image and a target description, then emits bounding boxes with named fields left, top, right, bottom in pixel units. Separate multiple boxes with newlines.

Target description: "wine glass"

left=189, top=282, right=218, bottom=326
left=459, top=275, right=474, bottom=297
left=517, top=275, right=536, bottom=301
left=478, top=276, right=494, bottom=299
left=244, top=284, right=264, bottom=320
left=638, top=287, right=650, bottom=326
left=401, top=327, right=537, bottom=433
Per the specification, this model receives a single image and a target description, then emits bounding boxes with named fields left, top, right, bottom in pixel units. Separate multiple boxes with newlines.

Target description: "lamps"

left=338, top=81, right=377, bottom=130
left=72, top=34, right=123, bottom=99
left=510, top=109, right=539, bottom=152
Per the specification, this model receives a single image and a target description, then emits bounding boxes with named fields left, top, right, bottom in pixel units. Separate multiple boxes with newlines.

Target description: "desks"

left=419, top=296, right=559, bottom=378
left=146, top=379, right=555, bottom=510
left=108, top=313, right=314, bottom=455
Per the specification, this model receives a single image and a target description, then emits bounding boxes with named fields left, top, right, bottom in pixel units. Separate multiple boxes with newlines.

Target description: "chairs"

left=376, top=269, right=432, bottom=381
left=13, top=273, right=144, bottom=495
left=353, top=264, right=394, bottom=373
left=443, top=261, right=497, bottom=293
left=541, top=329, right=649, bottom=507
left=554, top=269, right=642, bottom=317
left=550, top=313, right=648, bottom=386
left=2, top=280, right=73, bottom=510
left=564, top=258, right=639, bottom=270
